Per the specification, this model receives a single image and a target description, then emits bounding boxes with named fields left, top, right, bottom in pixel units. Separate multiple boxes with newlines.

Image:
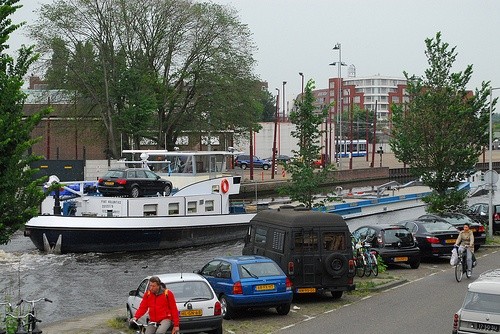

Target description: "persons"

left=454, top=223, right=474, bottom=277
left=129, top=276, right=179, bottom=334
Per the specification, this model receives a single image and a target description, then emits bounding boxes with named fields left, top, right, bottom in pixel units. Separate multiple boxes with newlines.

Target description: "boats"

left=22, top=149, right=257, bottom=253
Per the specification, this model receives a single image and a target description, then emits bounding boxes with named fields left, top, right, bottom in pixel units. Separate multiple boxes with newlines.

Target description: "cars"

left=193, top=255, right=293, bottom=320
left=312, top=153, right=328, bottom=168
left=419, top=212, right=487, bottom=253
left=466, top=203, right=500, bottom=236
left=125, top=271, right=226, bottom=334
left=95, top=167, right=173, bottom=199
left=352, top=224, right=420, bottom=269
left=392, top=218, right=461, bottom=264
left=262, top=155, right=295, bottom=162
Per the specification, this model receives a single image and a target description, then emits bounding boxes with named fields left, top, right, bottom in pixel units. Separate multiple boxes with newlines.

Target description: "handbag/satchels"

left=450, top=246, right=458, bottom=265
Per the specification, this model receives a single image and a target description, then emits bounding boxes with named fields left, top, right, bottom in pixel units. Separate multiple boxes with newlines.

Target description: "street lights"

left=299, top=72, right=304, bottom=107
left=488, top=85, right=500, bottom=240
left=283, top=81, right=287, bottom=124
left=329, top=41, right=344, bottom=140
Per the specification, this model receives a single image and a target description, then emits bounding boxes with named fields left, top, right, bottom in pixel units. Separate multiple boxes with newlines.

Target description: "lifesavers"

left=221, top=179, right=229, bottom=193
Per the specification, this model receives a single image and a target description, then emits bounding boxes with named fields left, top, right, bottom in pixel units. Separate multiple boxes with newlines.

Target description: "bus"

left=334, top=140, right=367, bottom=157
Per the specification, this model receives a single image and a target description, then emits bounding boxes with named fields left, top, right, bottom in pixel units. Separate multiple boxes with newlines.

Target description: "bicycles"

left=0, top=298, right=53, bottom=334
left=451, top=244, right=474, bottom=282
left=352, top=236, right=380, bottom=277
left=131, top=319, right=161, bottom=334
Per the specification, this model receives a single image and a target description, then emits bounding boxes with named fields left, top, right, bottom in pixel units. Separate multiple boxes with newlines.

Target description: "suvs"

left=234, top=154, right=273, bottom=171
left=242, top=206, right=356, bottom=300
